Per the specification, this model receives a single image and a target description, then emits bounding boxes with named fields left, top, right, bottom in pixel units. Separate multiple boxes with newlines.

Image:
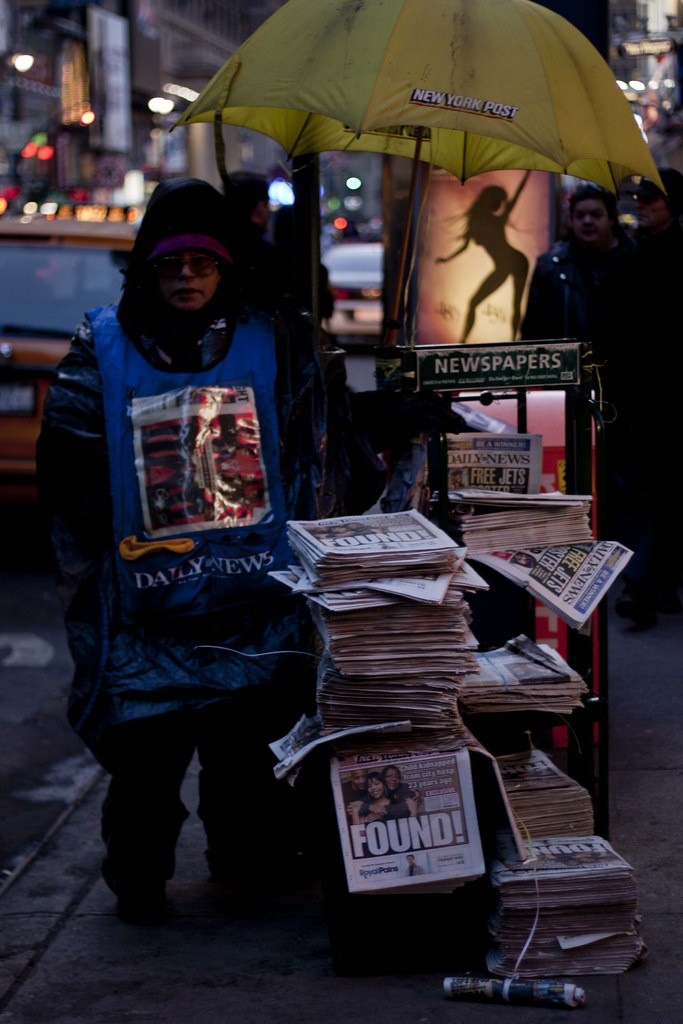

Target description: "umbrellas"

left=166, top=1, right=669, bottom=351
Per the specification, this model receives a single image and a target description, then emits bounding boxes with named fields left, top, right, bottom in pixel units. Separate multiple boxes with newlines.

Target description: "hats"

left=625, top=167, right=683, bottom=202
left=148, top=231, right=232, bottom=264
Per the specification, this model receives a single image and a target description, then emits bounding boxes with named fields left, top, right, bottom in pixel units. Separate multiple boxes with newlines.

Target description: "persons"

left=520, top=162, right=683, bottom=629
left=308, top=520, right=385, bottom=545
left=36, top=180, right=312, bottom=925
left=565, top=850, right=625, bottom=870
left=341, top=764, right=424, bottom=856
left=405, top=854, right=426, bottom=876
left=215, top=170, right=338, bottom=332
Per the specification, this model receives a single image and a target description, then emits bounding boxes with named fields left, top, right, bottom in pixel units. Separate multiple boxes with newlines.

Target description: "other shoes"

left=613, top=588, right=656, bottom=629
left=117, top=877, right=167, bottom=927
left=655, top=584, right=682, bottom=614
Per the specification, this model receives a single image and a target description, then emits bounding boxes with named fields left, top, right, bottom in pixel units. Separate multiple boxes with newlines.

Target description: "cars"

left=1, top=210, right=137, bottom=514
left=321, top=237, right=388, bottom=349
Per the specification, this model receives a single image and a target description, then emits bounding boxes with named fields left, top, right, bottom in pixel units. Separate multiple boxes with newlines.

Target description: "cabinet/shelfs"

left=376, top=338, right=613, bottom=839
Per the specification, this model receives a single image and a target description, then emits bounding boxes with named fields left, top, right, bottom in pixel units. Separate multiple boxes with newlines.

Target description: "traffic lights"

left=18, top=129, right=54, bottom=166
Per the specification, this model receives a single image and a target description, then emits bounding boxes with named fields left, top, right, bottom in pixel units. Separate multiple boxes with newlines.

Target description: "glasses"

left=153, top=252, right=220, bottom=278
left=633, top=193, right=671, bottom=205
